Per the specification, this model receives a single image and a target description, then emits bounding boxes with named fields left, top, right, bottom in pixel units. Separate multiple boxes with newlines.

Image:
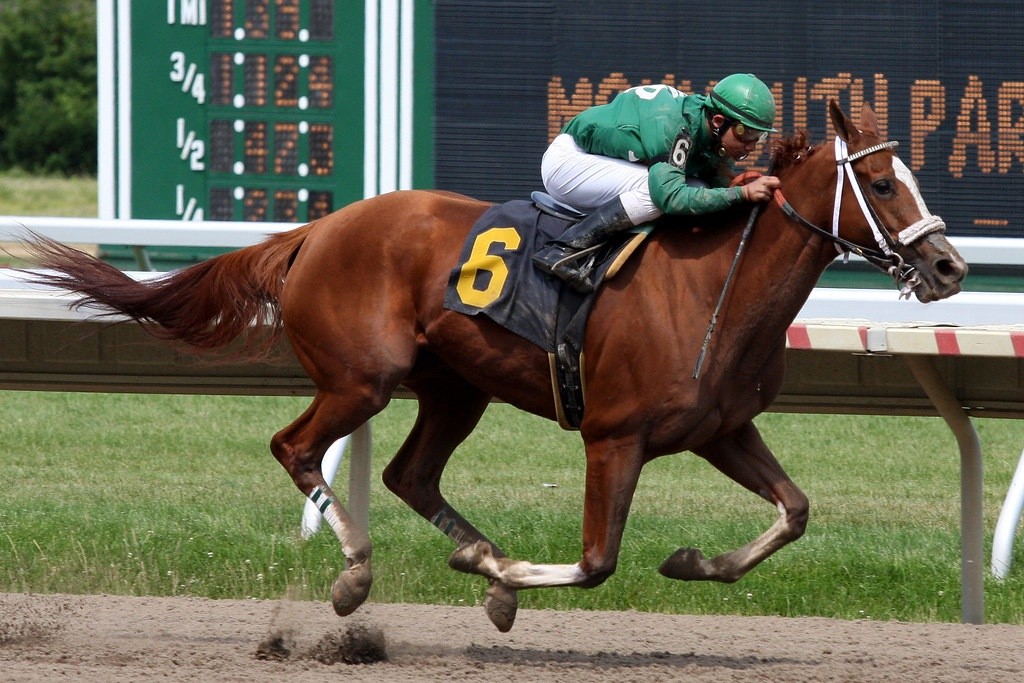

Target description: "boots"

left=531, top=194, right=636, bottom=287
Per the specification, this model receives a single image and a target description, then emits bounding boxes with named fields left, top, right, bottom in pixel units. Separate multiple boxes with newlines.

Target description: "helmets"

left=704, top=73, right=779, bottom=133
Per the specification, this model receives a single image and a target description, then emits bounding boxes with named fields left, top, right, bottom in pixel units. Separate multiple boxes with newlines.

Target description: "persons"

left=531, top=73, right=781, bottom=293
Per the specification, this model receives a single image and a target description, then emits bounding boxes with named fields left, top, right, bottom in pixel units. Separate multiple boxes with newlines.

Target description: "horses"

left=0, top=100, right=968, bottom=635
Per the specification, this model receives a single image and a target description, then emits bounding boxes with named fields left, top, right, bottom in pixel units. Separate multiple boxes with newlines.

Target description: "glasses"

left=732, top=121, right=767, bottom=144
